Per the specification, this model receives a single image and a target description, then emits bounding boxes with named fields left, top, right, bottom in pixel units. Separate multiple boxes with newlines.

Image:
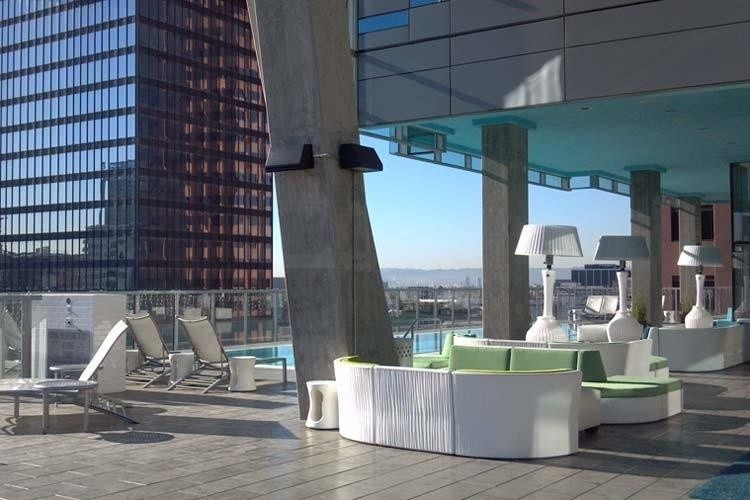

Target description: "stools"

left=305, top=381, right=337, bottom=428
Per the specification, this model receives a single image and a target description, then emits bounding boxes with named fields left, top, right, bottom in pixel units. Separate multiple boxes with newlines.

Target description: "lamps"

left=677, top=245, right=724, bottom=329
left=593, top=236, right=650, bottom=342
left=338, top=143, right=383, bottom=174
left=514, top=225, right=584, bottom=341
left=264, top=143, right=316, bottom=174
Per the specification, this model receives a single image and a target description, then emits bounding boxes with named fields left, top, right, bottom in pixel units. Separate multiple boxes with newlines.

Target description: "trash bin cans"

left=393, top=337, right=414, bottom=368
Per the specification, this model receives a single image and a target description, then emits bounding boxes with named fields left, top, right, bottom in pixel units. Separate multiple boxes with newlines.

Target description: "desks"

left=33, top=379, right=99, bottom=434
left=49, top=365, right=104, bottom=408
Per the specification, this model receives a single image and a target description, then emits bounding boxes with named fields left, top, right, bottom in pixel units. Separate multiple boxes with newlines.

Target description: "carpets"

left=689, top=450, right=750, bottom=500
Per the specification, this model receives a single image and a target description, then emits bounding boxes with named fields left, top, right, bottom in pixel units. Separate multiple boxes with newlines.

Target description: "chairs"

left=576, top=295, right=618, bottom=321
left=125, top=313, right=287, bottom=394
left=0, top=319, right=141, bottom=425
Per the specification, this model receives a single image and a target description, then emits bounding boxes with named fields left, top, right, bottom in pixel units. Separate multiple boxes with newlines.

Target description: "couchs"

left=334, top=333, right=684, bottom=459
left=577, top=320, right=750, bottom=372
